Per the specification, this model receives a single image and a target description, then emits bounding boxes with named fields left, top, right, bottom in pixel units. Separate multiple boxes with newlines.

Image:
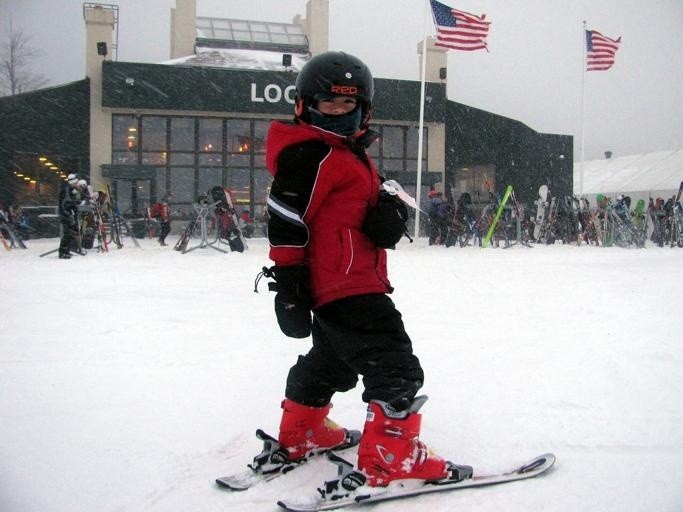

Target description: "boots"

left=276, top=394, right=350, bottom=462
left=353, top=394, right=475, bottom=490
left=57, top=247, right=73, bottom=260
left=156, top=239, right=168, bottom=247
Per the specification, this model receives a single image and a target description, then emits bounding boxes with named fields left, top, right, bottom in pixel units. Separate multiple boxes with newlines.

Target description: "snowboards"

left=212, top=186, right=247, bottom=252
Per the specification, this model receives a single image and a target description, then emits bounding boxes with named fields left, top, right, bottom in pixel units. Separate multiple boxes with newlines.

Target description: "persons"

left=265, top=50, right=472, bottom=483
left=1, top=172, right=255, bottom=259
left=429, top=190, right=682, bottom=249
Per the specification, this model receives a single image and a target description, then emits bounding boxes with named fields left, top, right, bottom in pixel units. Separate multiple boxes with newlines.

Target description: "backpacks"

left=149, top=202, right=167, bottom=219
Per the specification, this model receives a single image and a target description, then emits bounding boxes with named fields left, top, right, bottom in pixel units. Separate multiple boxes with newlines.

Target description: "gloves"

left=252, top=262, right=316, bottom=339
left=359, top=177, right=428, bottom=251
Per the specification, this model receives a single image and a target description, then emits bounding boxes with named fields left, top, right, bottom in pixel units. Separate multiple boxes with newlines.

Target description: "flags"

left=584, top=25, right=622, bottom=73
left=429, top=0, right=490, bottom=51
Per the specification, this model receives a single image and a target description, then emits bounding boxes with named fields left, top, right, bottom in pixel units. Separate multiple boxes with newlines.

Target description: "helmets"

left=65, top=173, right=78, bottom=185
left=75, top=179, right=87, bottom=187
left=290, top=48, right=377, bottom=137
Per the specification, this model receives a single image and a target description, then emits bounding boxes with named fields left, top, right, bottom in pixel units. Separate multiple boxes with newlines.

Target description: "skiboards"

left=447, top=184, right=682, bottom=250
left=216, top=450, right=555, bottom=512
left=88, top=184, right=122, bottom=253
